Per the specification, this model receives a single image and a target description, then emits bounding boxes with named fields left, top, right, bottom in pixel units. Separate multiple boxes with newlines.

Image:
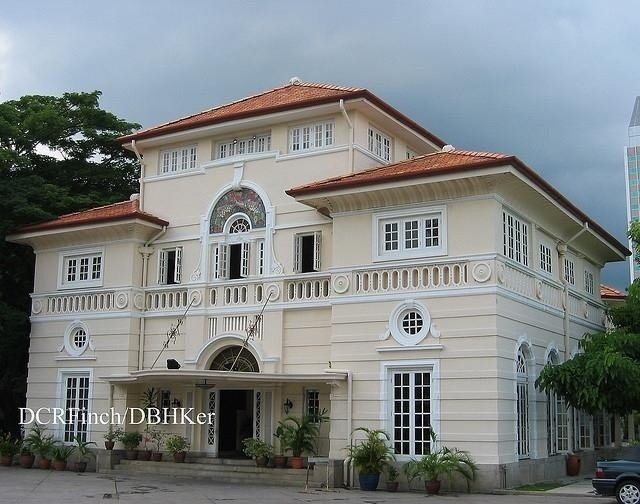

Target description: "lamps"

left=166, top=359, right=181, bottom=369
left=283, top=398, right=293, bottom=414
left=192, top=378, right=218, bottom=390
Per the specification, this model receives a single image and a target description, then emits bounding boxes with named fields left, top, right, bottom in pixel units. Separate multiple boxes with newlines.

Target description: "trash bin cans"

left=328, top=459, right=345, bottom=488
left=567, top=452, right=583, bottom=476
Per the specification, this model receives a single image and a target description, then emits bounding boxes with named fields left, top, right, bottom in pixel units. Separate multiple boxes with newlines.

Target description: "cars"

left=593, top=459, right=640, bottom=504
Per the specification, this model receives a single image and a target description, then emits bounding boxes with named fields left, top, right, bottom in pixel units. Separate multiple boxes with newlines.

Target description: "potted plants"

left=0, top=383, right=189, bottom=476
left=243, top=405, right=477, bottom=495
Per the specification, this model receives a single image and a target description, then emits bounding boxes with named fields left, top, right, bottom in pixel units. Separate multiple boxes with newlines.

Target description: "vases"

left=565, top=452, right=581, bottom=477
